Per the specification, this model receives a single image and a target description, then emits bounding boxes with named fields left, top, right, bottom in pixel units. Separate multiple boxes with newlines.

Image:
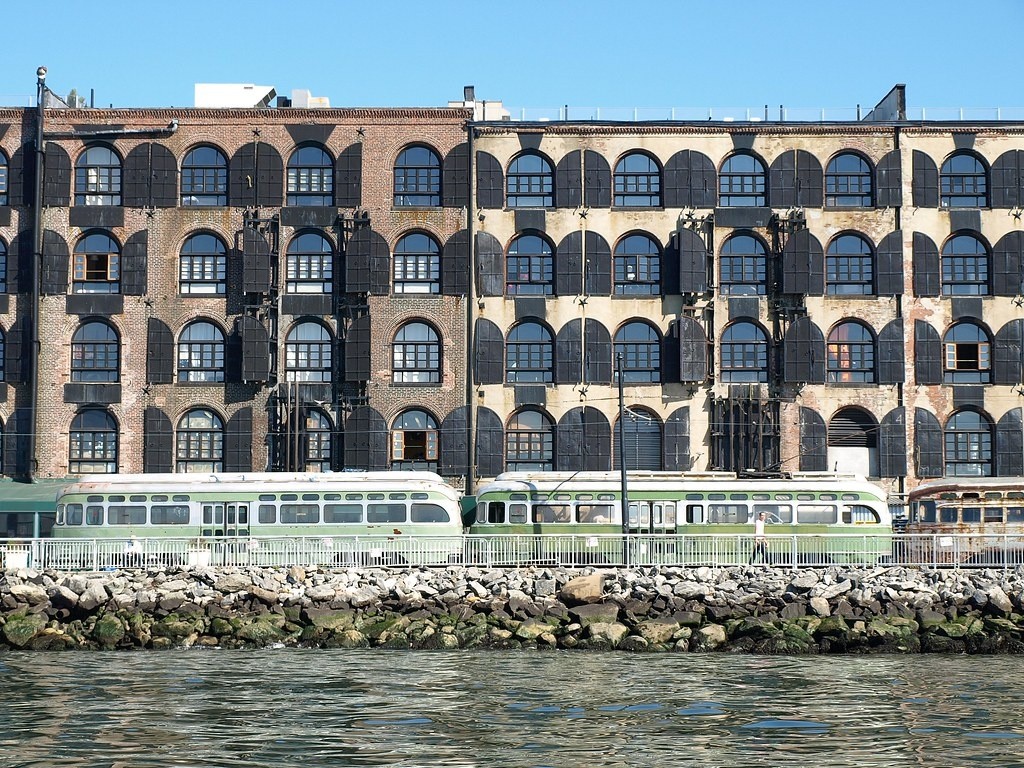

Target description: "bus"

left=470, top=470, right=893, bottom=569
left=50, top=473, right=464, bottom=568
left=903, top=478, right=1024, bottom=566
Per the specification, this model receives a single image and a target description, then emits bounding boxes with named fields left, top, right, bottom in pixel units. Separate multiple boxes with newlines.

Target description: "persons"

left=749, top=512, right=773, bottom=566
left=540, top=505, right=557, bottom=523
left=592, top=504, right=648, bottom=524
